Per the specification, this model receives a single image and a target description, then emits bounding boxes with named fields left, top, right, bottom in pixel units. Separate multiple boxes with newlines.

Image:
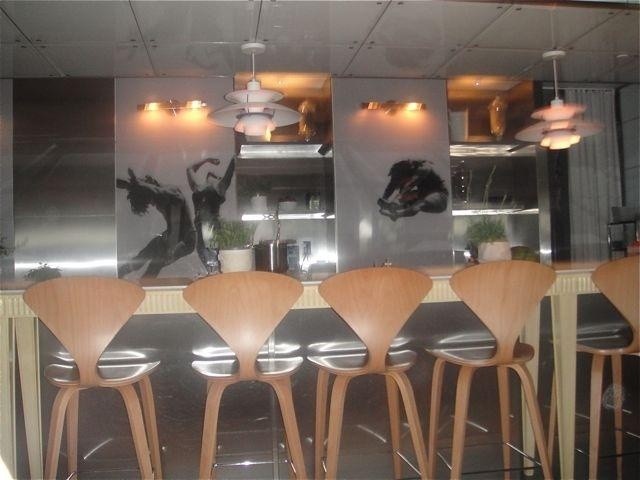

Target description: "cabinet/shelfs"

left=236, top=144, right=339, bottom=284
left=451, top=142, right=543, bottom=268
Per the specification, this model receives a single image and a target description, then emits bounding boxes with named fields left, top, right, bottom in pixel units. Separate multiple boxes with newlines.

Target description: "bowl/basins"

left=278, top=200, right=299, bottom=212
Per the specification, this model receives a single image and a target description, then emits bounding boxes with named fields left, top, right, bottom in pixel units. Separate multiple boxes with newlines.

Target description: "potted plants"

left=466, top=215, right=512, bottom=263
left=210, top=218, right=259, bottom=273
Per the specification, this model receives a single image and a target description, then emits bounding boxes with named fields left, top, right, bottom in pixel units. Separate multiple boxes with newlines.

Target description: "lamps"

left=137, top=99, right=207, bottom=114
left=361, top=100, right=427, bottom=115
left=206, top=44, right=303, bottom=138
left=513, top=51, right=605, bottom=150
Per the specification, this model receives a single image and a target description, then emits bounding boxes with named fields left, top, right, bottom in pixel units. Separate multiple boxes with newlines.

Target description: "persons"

left=185, top=153, right=234, bottom=274
left=116, top=166, right=196, bottom=288
left=376, top=159, right=449, bottom=222
